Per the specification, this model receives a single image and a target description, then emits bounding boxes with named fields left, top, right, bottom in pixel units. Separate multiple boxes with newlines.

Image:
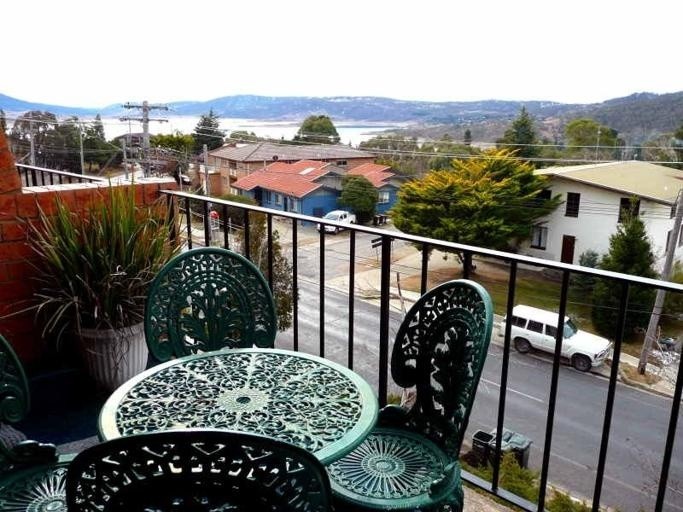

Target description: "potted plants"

left=0, top=166, right=193, bottom=389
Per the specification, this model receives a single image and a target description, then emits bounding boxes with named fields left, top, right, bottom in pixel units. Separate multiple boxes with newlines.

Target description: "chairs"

left=66, top=428, right=331, bottom=512
left=324, top=279, right=494, bottom=512
left=0, top=333, right=31, bottom=451
left=145, top=247, right=277, bottom=369
left=0, top=438, right=79, bottom=512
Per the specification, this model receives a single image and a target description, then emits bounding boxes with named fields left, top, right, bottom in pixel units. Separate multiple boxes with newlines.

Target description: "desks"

left=95, top=348, right=379, bottom=477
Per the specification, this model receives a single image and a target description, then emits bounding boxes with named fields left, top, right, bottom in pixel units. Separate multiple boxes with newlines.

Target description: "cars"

left=316, top=209, right=356, bottom=234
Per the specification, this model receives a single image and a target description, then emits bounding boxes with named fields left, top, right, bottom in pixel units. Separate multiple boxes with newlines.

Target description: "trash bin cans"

left=472, top=426, right=533, bottom=472
left=373, top=214, right=387, bottom=226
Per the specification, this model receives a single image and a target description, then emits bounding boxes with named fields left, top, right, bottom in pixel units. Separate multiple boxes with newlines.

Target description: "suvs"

left=498, top=304, right=614, bottom=372
